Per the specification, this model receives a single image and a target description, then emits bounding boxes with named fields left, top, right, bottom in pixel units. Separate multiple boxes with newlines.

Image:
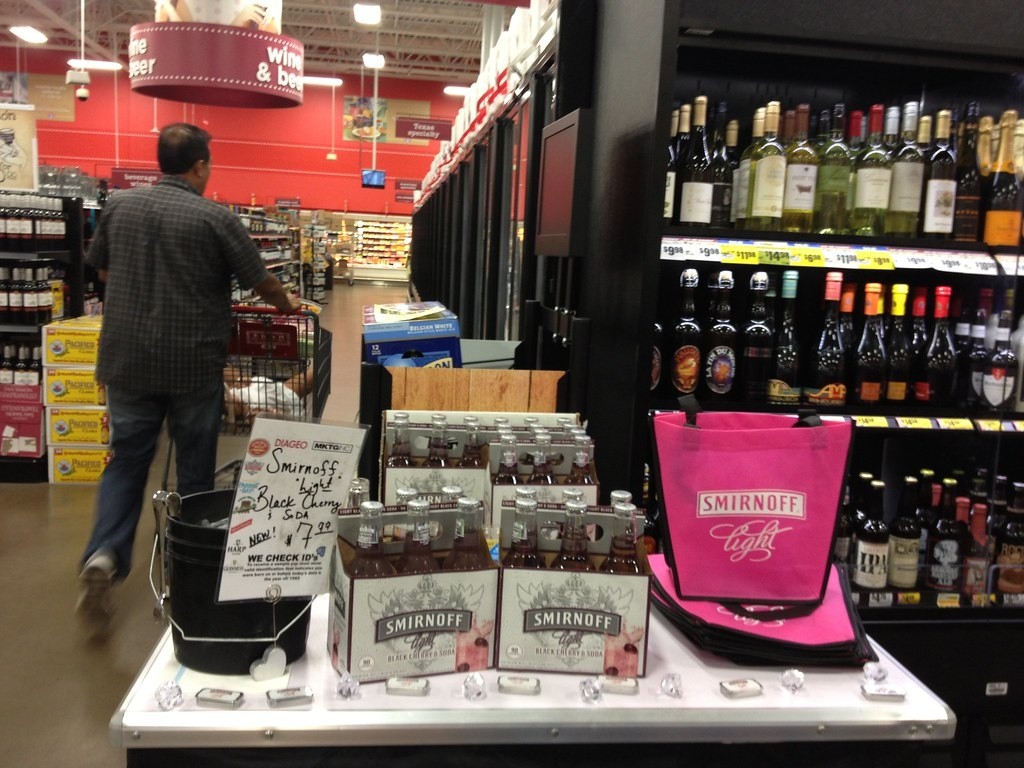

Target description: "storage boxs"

left=39, top=300, right=649, bottom=684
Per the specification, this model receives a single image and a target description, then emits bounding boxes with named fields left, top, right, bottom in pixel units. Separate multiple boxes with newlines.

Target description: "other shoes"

left=70, top=549, right=120, bottom=644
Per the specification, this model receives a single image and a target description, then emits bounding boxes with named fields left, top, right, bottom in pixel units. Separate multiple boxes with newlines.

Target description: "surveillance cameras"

left=75, top=89, right=89, bottom=102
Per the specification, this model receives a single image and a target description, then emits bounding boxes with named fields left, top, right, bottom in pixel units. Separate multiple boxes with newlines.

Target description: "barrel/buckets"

left=148, top=489, right=318, bottom=676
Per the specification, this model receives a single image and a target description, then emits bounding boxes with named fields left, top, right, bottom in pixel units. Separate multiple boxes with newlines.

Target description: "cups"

left=38, top=163, right=98, bottom=205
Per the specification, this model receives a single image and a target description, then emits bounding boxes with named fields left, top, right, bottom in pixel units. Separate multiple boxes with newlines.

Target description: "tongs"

left=151, top=490, right=183, bottom=627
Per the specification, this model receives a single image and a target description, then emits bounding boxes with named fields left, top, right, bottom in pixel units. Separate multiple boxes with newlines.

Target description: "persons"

left=77, top=121, right=301, bottom=632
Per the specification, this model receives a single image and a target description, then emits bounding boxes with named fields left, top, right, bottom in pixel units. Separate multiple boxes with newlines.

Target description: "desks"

left=106, top=556, right=958, bottom=768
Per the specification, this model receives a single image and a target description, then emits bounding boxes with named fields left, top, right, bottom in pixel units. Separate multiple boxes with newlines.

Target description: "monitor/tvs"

left=361, top=170, right=386, bottom=189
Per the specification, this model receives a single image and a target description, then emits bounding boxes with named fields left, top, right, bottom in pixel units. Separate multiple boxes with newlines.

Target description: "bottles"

left=346, top=92, right=1024, bottom=615
left=0, top=192, right=71, bottom=387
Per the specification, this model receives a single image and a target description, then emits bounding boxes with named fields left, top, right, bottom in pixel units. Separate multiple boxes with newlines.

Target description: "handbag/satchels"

left=653, top=394, right=858, bottom=620
left=647, top=550, right=879, bottom=672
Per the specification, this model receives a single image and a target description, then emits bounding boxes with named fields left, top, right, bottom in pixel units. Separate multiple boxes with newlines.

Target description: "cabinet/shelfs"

left=591, top=19, right=1024, bottom=626
left=0, top=194, right=413, bottom=484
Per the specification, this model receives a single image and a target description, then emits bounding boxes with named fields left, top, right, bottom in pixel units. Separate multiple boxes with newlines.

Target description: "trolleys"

left=160, top=301, right=332, bottom=494
